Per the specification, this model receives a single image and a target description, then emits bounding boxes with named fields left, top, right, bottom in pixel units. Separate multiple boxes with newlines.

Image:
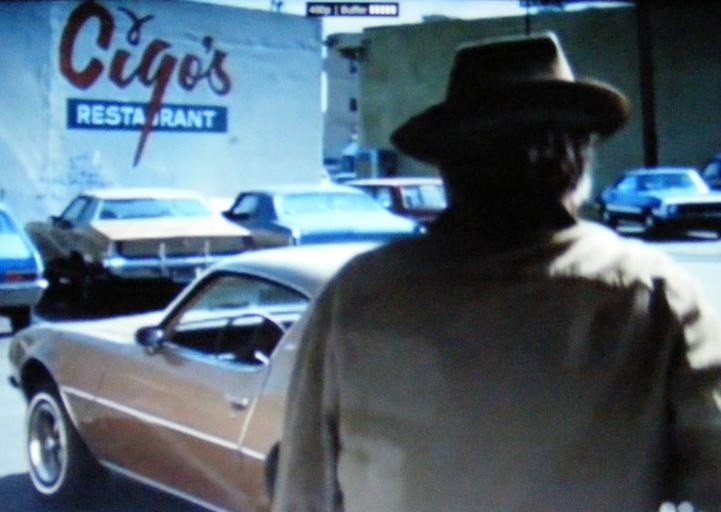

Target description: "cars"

left=23, top=186, right=255, bottom=317
left=346, top=176, right=446, bottom=227
left=3, top=243, right=386, bottom=511
left=0, top=204, right=49, bottom=329
left=705, top=153, right=720, bottom=190
left=594, top=164, right=720, bottom=242
left=220, top=181, right=429, bottom=252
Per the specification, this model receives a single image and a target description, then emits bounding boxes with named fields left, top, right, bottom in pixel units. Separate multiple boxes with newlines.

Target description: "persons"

left=271, top=32, right=721, bottom=510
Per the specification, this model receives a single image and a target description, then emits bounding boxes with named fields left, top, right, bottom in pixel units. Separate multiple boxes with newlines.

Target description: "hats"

left=387, top=27, right=633, bottom=168
